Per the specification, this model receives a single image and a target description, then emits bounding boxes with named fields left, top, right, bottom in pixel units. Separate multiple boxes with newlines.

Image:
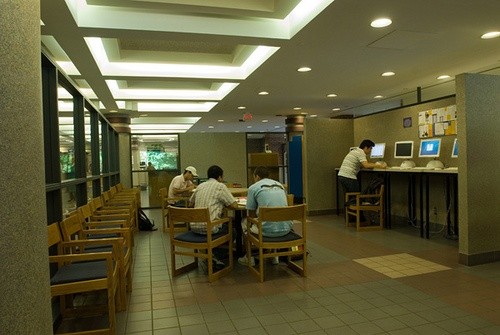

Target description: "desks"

left=231, top=197, right=252, bottom=256
left=189, top=187, right=249, bottom=196
left=334, top=166, right=458, bottom=243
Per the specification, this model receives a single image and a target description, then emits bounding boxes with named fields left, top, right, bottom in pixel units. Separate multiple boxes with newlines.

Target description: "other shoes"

left=269, top=256, right=278, bottom=263
left=238, top=254, right=255, bottom=267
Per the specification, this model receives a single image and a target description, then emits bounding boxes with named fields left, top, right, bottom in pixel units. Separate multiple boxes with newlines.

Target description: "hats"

left=185, top=166, right=198, bottom=177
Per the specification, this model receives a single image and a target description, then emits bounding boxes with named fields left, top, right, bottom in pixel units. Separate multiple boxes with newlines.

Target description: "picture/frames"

left=403, top=117, right=412, bottom=127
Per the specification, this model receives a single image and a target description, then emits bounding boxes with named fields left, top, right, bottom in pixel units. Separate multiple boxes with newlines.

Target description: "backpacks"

left=137, top=207, right=158, bottom=231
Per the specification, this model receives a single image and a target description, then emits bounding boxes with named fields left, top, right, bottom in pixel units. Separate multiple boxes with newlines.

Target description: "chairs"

left=346, top=182, right=385, bottom=231
left=159, top=186, right=308, bottom=283
left=47, top=184, right=142, bottom=335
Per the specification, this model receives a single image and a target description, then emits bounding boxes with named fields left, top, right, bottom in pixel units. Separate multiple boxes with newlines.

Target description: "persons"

left=167, top=166, right=199, bottom=207
left=188, top=165, right=237, bottom=250
left=238, top=166, right=292, bottom=266
left=146, top=162, right=155, bottom=170
left=337, top=140, right=387, bottom=227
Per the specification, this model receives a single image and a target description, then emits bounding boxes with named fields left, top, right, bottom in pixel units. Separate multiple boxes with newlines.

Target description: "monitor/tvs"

left=394, top=141, right=416, bottom=168
left=451, top=138, right=458, bottom=158
left=418, top=138, right=444, bottom=168
left=370, top=143, right=387, bottom=167
